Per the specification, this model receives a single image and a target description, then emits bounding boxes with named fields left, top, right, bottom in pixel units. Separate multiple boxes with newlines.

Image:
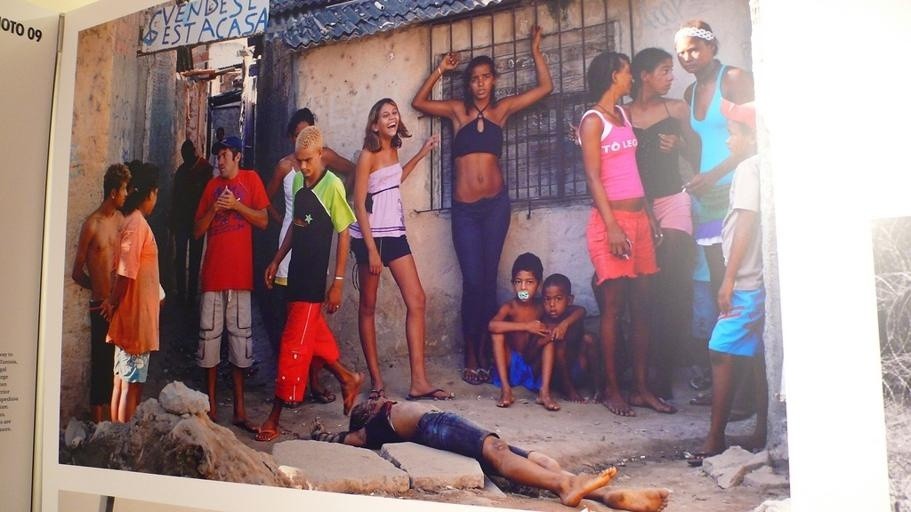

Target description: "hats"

left=212, top=136, right=242, bottom=155
left=720, top=96, right=756, bottom=130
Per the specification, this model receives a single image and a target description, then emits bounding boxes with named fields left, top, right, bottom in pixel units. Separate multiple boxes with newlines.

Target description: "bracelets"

left=438, top=67, right=442, bottom=76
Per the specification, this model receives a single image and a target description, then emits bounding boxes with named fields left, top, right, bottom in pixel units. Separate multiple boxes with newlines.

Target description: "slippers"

left=368, top=388, right=385, bottom=400
left=406, top=389, right=456, bottom=400
left=690, top=369, right=714, bottom=404
left=283, top=371, right=364, bottom=415
left=687, top=451, right=708, bottom=464
left=255, top=426, right=280, bottom=441
left=462, top=368, right=494, bottom=384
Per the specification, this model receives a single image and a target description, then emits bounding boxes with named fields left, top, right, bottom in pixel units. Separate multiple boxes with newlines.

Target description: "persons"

left=570, top=23, right=769, bottom=468
left=489, top=251, right=606, bottom=411
left=69, top=160, right=164, bottom=424
left=166, top=140, right=213, bottom=304
left=254, top=107, right=365, bottom=442
left=194, top=134, right=272, bottom=434
left=411, top=25, right=554, bottom=384
left=350, top=98, right=453, bottom=403
left=210, top=127, right=229, bottom=169
left=302, top=397, right=672, bottom=510
